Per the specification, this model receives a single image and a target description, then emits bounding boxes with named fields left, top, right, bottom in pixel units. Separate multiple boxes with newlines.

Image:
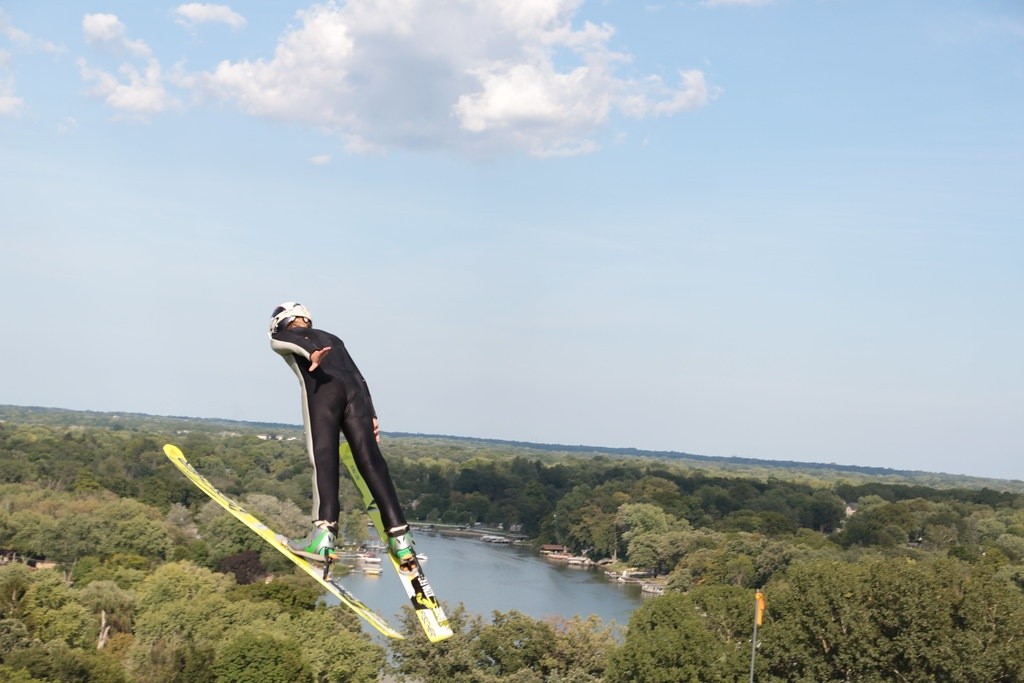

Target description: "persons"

left=266, top=299, right=421, bottom=567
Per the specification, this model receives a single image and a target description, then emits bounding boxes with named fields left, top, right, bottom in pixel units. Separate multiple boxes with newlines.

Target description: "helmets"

left=269, top=302, right=313, bottom=336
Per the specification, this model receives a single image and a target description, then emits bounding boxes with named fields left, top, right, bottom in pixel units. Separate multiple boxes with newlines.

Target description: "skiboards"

left=161, top=437, right=458, bottom=646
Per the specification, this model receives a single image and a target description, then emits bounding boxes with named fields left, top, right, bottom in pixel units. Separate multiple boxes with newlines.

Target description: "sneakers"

left=386, top=524, right=419, bottom=574
left=286, top=522, right=339, bottom=563
